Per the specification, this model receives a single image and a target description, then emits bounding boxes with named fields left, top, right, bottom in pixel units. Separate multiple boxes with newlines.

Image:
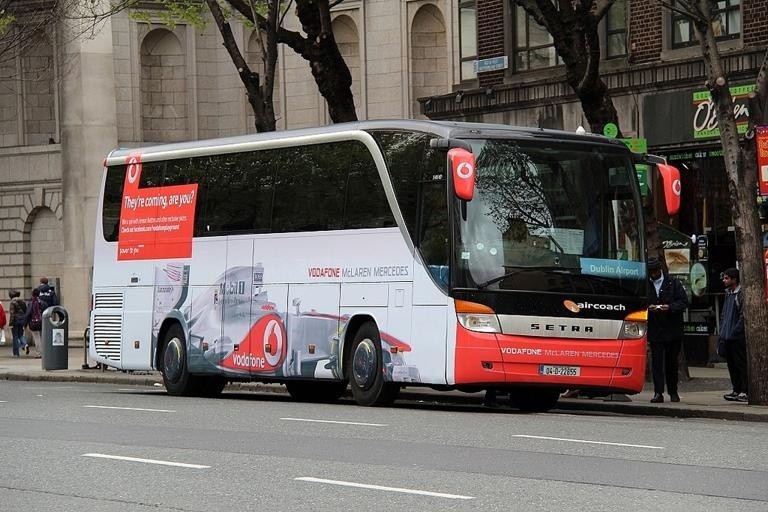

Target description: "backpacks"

left=29, top=296, right=42, bottom=331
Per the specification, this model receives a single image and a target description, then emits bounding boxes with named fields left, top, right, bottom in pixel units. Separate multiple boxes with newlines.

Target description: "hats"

left=647, top=256, right=662, bottom=269
left=40, top=277, right=48, bottom=283
left=32, top=288, right=40, bottom=297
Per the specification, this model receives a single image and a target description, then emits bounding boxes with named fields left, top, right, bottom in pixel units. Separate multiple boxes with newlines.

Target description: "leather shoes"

left=560, top=388, right=580, bottom=399
left=649, top=392, right=664, bottom=403
left=668, top=392, right=681, bottom=402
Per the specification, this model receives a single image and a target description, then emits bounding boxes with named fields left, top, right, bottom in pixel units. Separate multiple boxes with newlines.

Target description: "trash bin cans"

left=42, top=306, right=68, bottom=370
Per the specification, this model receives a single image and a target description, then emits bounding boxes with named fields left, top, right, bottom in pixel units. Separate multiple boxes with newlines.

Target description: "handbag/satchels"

left=709, top=336, right=726, bottom=363
left=0, top=328, right=6, bottom=346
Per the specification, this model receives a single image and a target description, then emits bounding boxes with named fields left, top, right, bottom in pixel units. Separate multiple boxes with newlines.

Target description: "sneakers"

left=724, top=390, right=748, bottom=402
left=24, top=344, right=29, bottom=355
left=33, top=352, right=41, bottom=358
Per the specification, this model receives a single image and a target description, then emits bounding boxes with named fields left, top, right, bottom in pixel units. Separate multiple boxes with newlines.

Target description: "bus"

left=89, top=116, right=683, bottom=407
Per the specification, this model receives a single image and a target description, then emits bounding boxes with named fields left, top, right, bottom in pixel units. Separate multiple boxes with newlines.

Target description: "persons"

left=719, top=268, right=748, bottom=402
left=22, top=287, right=49, bottom=358
left=0, top=303, right=7, bottom=329
left=647, top=256, right=688, bottom=403
left=9, top=289, right=30, bottom=358
left=39, top=278, right=58, bottom=306
